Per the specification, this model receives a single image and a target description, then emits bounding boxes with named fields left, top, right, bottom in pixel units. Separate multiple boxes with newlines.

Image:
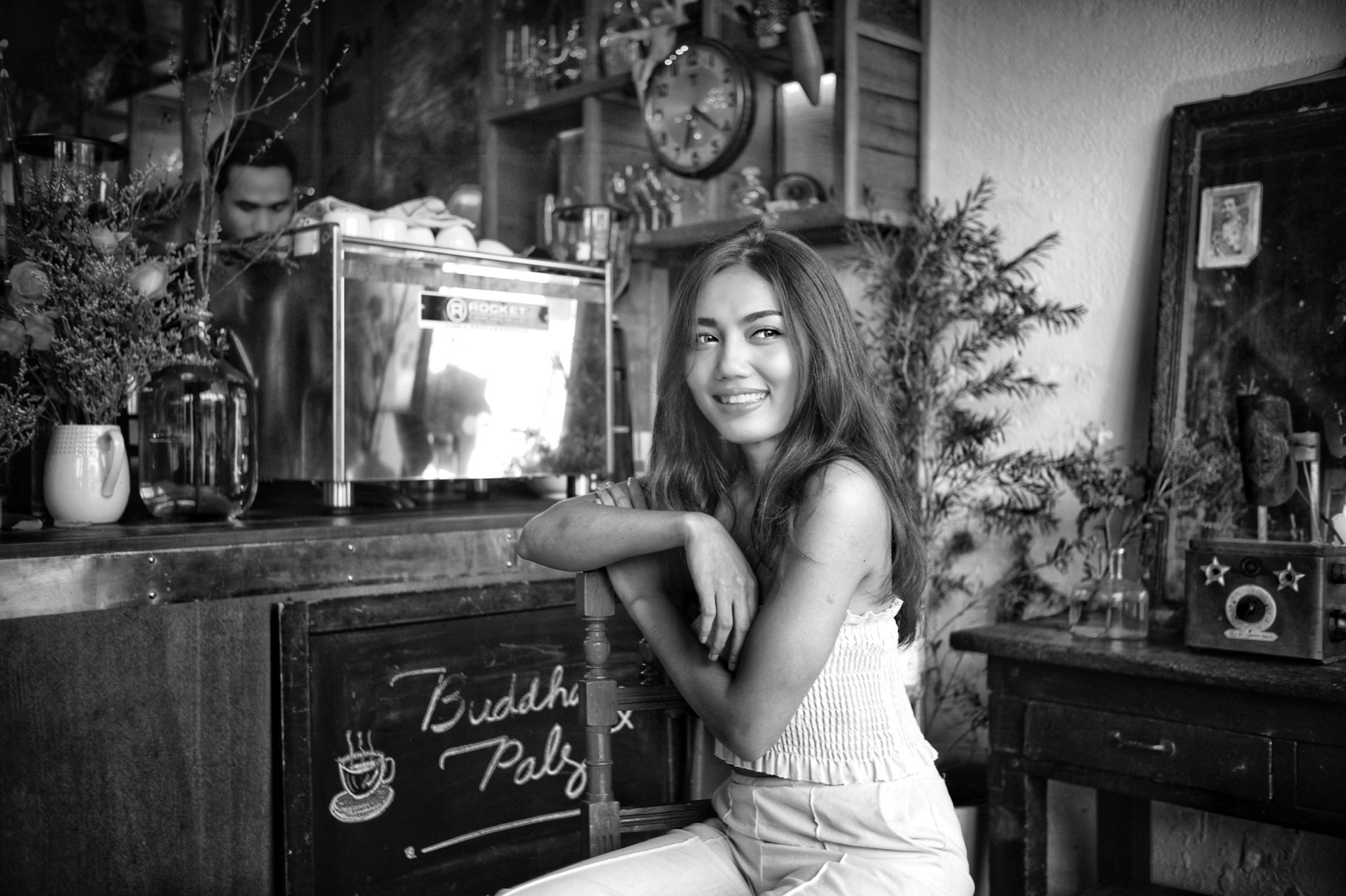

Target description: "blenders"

left=546, top=202, right=635, bottom=489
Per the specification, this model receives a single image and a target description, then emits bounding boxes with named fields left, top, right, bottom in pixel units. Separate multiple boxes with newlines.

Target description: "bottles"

left=731, top=166, right=769, bottom=217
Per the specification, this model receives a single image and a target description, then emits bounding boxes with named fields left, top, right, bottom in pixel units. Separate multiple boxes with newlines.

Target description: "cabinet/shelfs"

left=479, top=0, right=929, bottom=243
left=948, top=606, right=1345, bottom=896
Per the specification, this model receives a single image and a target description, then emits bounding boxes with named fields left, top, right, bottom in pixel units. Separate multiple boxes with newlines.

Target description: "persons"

left=514, top=232, right=975, bottom=896
left=205, top=120, right=300, bottom=238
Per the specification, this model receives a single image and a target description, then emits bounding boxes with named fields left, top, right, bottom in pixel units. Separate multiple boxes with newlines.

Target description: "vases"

left=1069, top=546, right=1153, bottom=641
left=140, top=308, right=260, bottom=524
left=42, top=424, right=129, bottom=528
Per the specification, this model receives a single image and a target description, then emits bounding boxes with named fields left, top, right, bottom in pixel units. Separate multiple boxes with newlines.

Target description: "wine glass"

left=604, top=161, right=700, bottom=230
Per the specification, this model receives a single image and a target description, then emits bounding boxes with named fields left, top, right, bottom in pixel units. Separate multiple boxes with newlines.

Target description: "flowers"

left=0, top=164, right=228, bottom=467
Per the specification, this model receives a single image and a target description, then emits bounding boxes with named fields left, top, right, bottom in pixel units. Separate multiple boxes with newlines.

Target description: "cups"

left=501, top=16, right=583, bottom=99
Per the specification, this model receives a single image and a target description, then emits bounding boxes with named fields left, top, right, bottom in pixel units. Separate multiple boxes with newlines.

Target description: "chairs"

left=574, top=569, right=718, bottom=853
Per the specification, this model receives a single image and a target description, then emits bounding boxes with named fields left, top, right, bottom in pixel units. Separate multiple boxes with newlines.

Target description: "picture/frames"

left=1066, top=418, right=1226, bottom=623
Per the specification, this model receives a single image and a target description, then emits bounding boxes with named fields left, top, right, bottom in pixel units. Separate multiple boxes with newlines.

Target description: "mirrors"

left=1138, top=65, right=1345, bottom=607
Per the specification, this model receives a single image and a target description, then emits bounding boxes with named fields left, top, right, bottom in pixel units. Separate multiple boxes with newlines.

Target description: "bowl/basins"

left=527, top=473, right=597, bottom=499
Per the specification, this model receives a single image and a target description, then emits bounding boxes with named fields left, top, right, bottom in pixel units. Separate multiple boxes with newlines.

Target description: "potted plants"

left=837, top=167, right=1088, bottom=896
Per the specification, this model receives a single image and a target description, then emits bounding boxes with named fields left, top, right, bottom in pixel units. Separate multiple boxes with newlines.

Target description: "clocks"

left=644, top=35, right=757, bottom=181
